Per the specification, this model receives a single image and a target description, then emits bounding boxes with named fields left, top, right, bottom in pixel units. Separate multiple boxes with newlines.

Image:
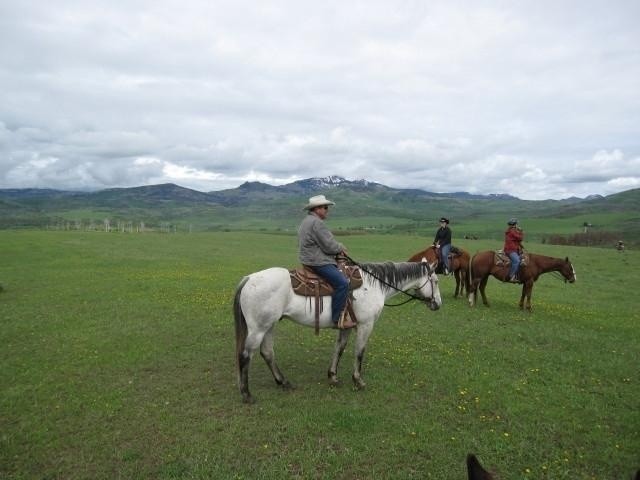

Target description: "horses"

left=233, top=256, right=442, bottom=405
left=404, top=245, right=471, bottom=298
left=468, top=250, right=576, bottom=313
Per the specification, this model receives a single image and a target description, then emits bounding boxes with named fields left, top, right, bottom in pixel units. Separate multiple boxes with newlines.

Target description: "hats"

left=438, top=217, right=449, bottom=225
left=507, top=217, right=518, bottom=225
left=303, top=193, right=339, bottom=212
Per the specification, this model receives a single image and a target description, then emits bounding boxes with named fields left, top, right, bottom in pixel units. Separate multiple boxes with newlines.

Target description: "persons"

left=505, top=217, right=525, bottom=283
left=431, top=217, right=453, bottom=276
left=298, top=194, right=357, bottom=329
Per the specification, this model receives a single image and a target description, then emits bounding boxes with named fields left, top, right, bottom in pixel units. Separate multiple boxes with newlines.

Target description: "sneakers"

left=508, top=274, right=522, bottom=284
left=334, top=319, right=358, bottom=329
